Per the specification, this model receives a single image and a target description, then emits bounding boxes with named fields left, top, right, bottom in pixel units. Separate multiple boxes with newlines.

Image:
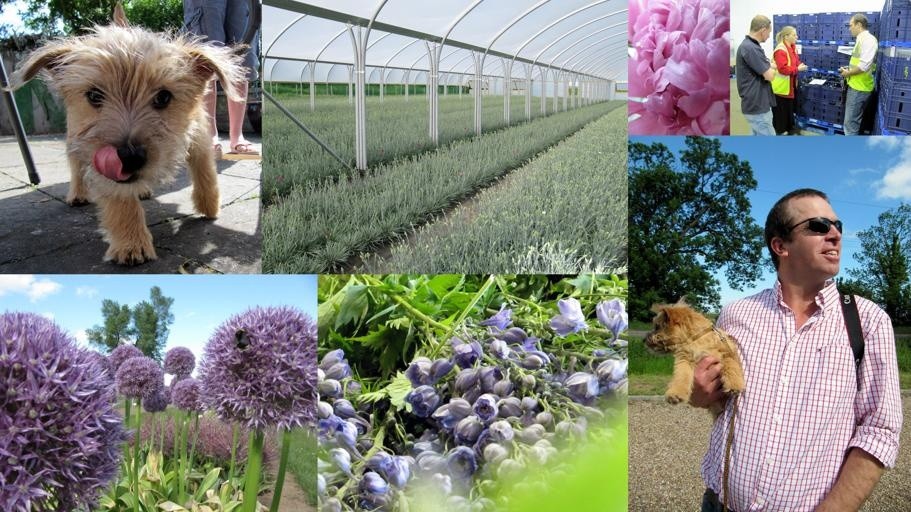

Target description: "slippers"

left=214, top=141, right=261, bottom=155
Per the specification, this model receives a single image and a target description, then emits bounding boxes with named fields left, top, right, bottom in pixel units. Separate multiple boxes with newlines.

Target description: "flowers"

left=628, top=0, right=730, bottom=135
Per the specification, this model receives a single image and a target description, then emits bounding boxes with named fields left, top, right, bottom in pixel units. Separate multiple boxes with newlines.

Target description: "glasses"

left=783, top=216, right=843, bottom=242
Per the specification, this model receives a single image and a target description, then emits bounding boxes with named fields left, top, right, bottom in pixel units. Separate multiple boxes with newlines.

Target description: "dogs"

left=641, top=294, right=746, bottom=421
left=1, top=2, right=252, bottom=269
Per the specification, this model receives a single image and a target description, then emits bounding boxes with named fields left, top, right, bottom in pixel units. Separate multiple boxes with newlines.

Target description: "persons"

left=736, top=14, right=777, bottom=135
left=837, top=13, right=879, bottom=136
left=701, top=188, right=904, bottom=512
left=770, top=25, right=808, bottom=134
